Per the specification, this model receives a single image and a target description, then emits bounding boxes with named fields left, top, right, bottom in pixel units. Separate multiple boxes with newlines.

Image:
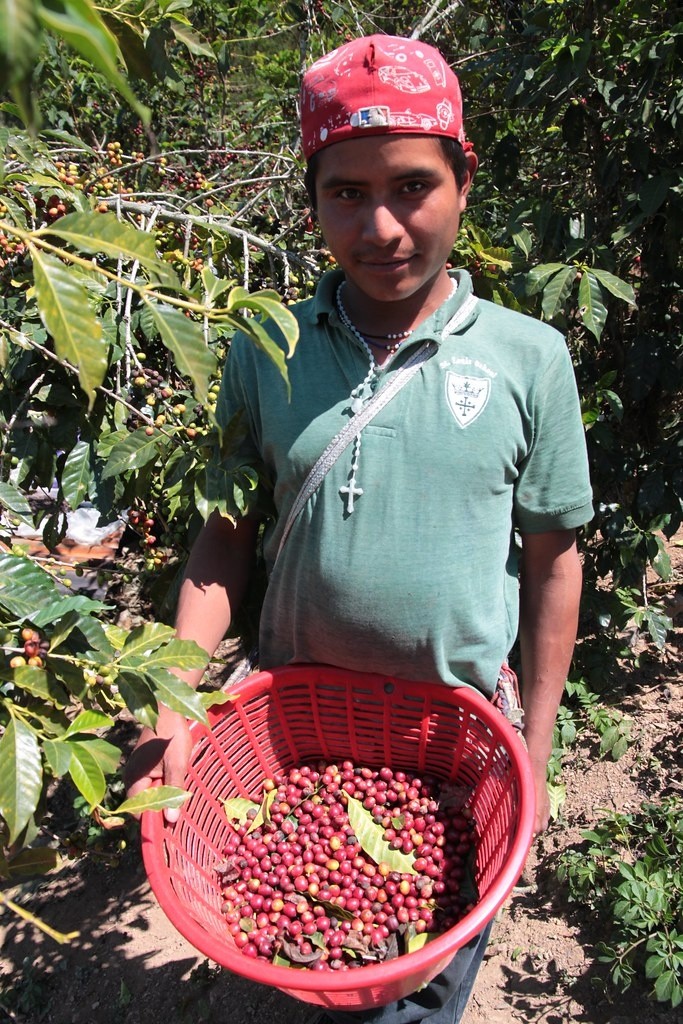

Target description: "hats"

left=301, top=34, right=467, bottom=157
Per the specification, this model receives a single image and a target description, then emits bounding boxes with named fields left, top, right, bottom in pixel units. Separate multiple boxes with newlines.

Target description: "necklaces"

left=333, top=277, right=458, bottom=516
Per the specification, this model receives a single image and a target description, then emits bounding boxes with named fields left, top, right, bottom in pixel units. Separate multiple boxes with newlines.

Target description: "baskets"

left=141, top=665, right=534, bottom=1010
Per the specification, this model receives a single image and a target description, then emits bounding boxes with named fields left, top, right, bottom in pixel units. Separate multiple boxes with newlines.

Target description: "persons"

left=126, top=36, right=592, bottom=1024
